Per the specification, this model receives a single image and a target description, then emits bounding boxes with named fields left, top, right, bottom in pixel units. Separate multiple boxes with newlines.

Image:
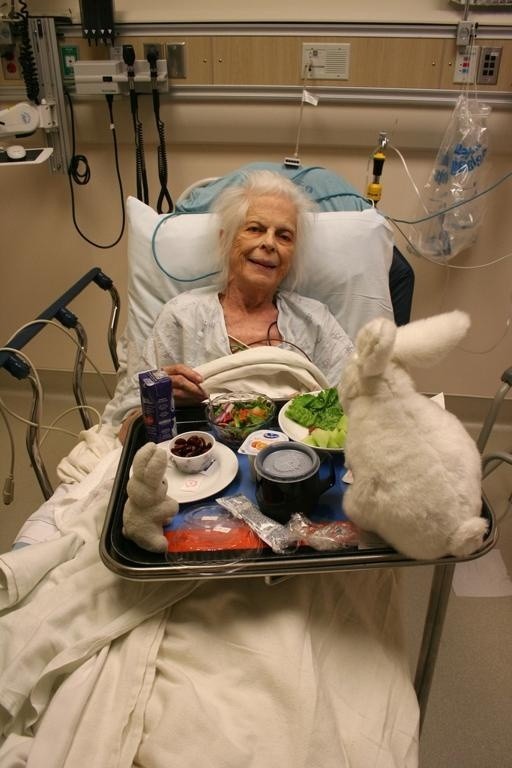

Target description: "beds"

left=0, top=154, right=502, bottom=767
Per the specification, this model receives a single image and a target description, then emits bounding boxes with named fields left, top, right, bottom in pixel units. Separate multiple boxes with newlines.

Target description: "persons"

left=112, top=169, right=380, bottom=447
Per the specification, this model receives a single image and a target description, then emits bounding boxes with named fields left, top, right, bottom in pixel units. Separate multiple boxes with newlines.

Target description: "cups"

left=250, top=441, right=338, bottom=519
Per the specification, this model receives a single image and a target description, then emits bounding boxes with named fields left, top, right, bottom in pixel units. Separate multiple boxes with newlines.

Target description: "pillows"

left=109, top=195, right=397, bottom=379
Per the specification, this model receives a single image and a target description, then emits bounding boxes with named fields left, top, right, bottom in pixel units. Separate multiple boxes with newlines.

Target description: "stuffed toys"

left=123, top=440, right=182, bottom=556
left=340, top=309, right=489, bottom=557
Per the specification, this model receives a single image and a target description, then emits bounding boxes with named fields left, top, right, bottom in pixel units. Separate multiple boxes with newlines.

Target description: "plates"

left=128, top=440, right=240, bottom=507
left=278, top=387, right=345, bottom=453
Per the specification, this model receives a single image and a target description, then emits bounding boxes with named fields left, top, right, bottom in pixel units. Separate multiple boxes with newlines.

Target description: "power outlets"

left=142, top=41, right=169, bottom=67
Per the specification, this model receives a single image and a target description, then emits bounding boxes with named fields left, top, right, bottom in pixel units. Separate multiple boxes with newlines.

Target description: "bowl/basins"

left=205, top=391, right=276, bottom=440
left=163, top=504, right=264, bottom=575
left=169, top=428, right=217, bottom=472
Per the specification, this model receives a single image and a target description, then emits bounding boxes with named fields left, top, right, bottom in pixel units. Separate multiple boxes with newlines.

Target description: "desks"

left=92, top=403, right=497, bottom=580
